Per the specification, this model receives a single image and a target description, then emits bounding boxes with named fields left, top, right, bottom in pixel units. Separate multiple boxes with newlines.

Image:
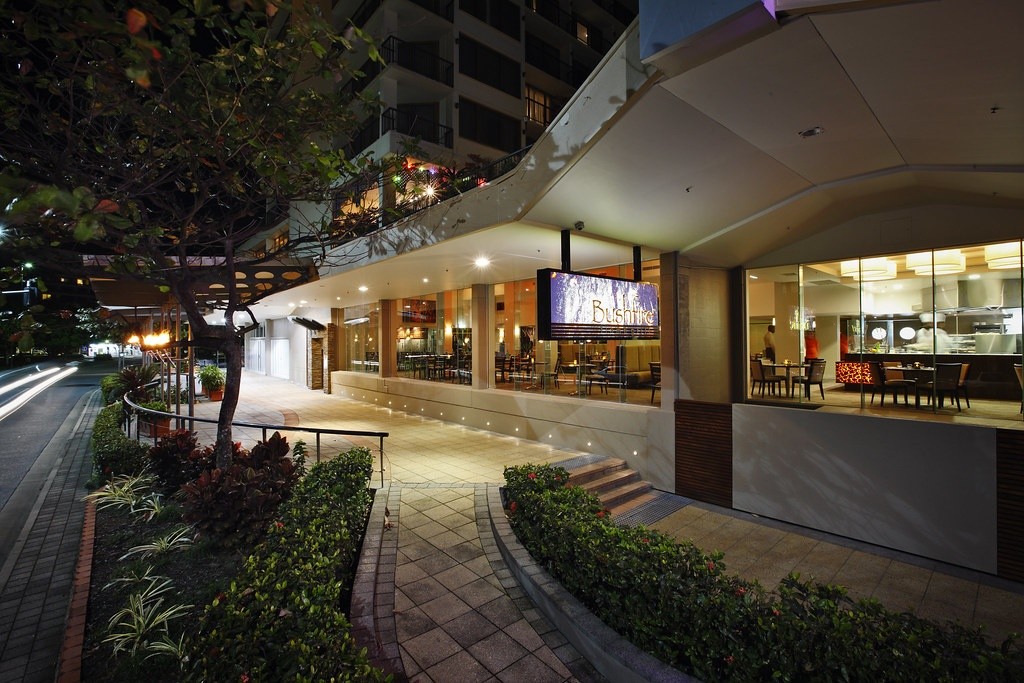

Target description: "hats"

left=920, top=313, right=933, bottom=322
left=937, top=313, right=946, bottom=322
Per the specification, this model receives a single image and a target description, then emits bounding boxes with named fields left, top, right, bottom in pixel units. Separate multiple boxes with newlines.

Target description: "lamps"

left=985, top=241, right=1024, bottom=262
left=905, top=250, right=961, bottom=270
left=853, top=262, right=897, bottom=281
left=988, top=261, right=1023, bottom=269
left=841, top=258, right=887, bottom=277
left=915, top=255, right=966, bottom=276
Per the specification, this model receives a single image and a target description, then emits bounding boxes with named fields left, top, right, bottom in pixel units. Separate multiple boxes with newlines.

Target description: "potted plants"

left=143, top=403, right=175, bottom=437
left=196, top=365, right=226, bottom=401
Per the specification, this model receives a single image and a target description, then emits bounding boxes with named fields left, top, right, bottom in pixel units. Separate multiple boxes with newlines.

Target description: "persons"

left=764, top=325, right=776, bottom=375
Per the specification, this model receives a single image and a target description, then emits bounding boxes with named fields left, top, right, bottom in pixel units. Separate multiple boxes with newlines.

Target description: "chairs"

left=648, top=362, right=662, bottom=404
left=915, top=363, right=962, bottom=412
left=586, top=359, right=610, bottom=395
left=756, top=357, right=787, bottom=397
left=881, top=362, right=916, bottom=405
left=868, top=362, right=908, bottom=406
left=541, top=357, right=565, bottom=389
left=791, top=358, right=825, bottom=398
left=926, top=363, right=971, bottom=408
left=1013, top=364, right=1023, bottom=414
left=750, top=360, right=781, bottom=399
left=792, top=361, right=826, bottom=401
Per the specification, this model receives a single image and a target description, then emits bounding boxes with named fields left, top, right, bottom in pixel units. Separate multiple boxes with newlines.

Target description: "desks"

left=762, top=363, right=810, bottom=398
left=883, top=366, right=935, bottom=410
left=519, top=362, right=548, bottom=390
left=590, top=359, right=615, bottom=371
left=562, top=363, right=595, bottom=395
